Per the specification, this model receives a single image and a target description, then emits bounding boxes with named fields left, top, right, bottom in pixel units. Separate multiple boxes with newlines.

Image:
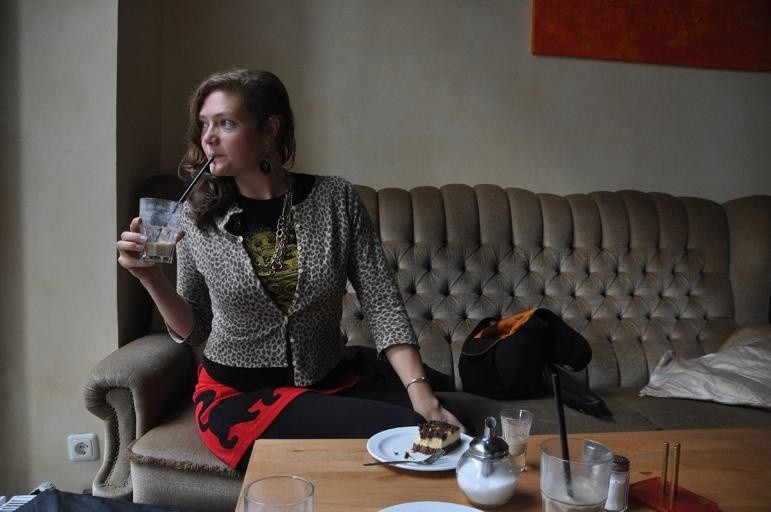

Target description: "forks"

left=363, top=449, right=445, bottom=466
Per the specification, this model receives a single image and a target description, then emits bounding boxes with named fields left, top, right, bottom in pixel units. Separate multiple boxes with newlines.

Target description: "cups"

left=244, top=476, right=314, bottom=512
left=139, top=197, right=183, bottom=264
left=540, top=437, right=614, bottom=511
left=500, top=408, right=533, bottom=471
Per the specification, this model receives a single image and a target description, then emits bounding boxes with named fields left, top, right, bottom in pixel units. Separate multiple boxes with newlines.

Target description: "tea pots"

left=456, top=417, right=519, bottom=507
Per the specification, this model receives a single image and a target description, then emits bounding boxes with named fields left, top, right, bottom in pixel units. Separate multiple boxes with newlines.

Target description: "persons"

left=116, top=69, right=476, bottom=475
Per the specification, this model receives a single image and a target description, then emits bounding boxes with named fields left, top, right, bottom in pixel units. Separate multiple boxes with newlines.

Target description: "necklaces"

left=252, top=174, right=295, bottom=273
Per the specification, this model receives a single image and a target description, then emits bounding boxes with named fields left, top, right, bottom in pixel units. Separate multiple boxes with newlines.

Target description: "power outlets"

left=68, top=433, right=100, bottom=462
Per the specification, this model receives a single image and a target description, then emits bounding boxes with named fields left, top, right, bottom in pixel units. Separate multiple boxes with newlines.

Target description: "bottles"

left=604, top=455, right=629, bottom=512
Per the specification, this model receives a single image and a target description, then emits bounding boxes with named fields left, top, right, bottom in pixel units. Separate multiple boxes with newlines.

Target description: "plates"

left=376, top=501, right=484, bottom=512
left=366, top=426, right=474, bottom=471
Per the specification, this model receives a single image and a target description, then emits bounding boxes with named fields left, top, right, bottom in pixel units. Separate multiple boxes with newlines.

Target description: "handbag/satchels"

left=459, top=308, right=592, bottom=400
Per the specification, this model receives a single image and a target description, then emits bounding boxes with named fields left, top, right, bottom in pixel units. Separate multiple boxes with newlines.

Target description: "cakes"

left=412, top=420, right=460, bottom=457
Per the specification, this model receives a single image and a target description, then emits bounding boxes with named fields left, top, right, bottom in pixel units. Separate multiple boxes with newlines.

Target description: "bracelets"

left=406, top=377, right=429, bottom=388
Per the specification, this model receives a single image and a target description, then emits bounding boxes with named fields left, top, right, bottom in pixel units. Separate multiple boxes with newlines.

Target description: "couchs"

left=82, top=183, right=771, bottom=511
